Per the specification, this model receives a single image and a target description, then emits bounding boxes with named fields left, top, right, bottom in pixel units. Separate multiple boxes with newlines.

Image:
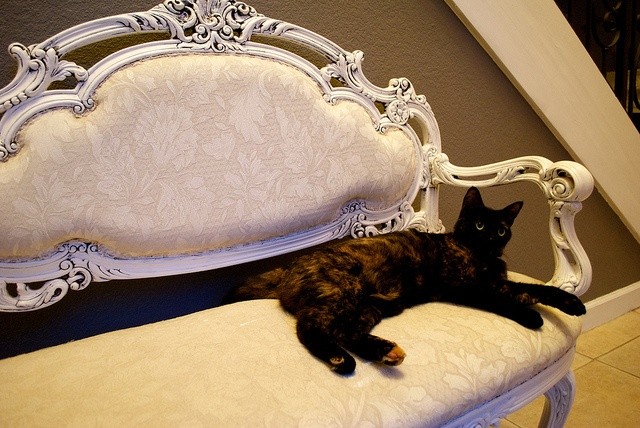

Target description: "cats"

left=225, top=186, right=586, bottom=376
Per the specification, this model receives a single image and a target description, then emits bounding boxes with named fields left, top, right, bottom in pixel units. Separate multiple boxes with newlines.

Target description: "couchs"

left=1, top=0, right=595, bottom=428
